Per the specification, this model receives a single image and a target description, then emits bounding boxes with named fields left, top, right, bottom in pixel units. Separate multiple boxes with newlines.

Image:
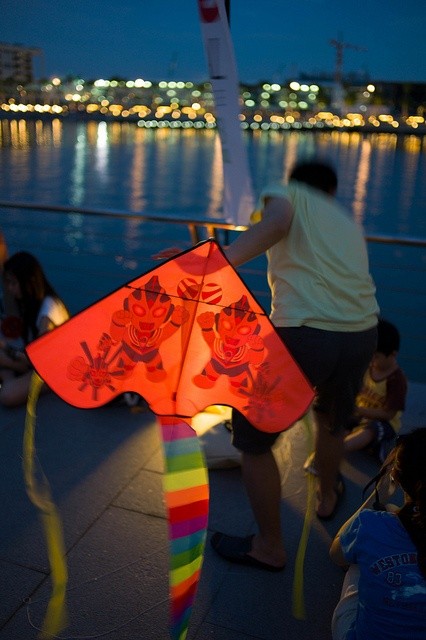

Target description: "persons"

left=301, top=317, right=409, bottom=478
left=151, top=150, right=385, bottom=575
left=0, top=236, right=75, bottom=409
left=328, top=425, right=426, bottom=640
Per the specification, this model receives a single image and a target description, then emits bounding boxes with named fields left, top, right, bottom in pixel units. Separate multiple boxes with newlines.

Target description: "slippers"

left=210, top=531, right=286, bottom=574
left=317, top=470, right=347, bottom=519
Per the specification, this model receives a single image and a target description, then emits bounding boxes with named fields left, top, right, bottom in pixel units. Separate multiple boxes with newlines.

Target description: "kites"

left=17, top=239, right=322, bottom=640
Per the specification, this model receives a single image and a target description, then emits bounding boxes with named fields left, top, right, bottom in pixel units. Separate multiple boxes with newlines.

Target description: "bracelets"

left=375, top=487, right=380, bottom=505
